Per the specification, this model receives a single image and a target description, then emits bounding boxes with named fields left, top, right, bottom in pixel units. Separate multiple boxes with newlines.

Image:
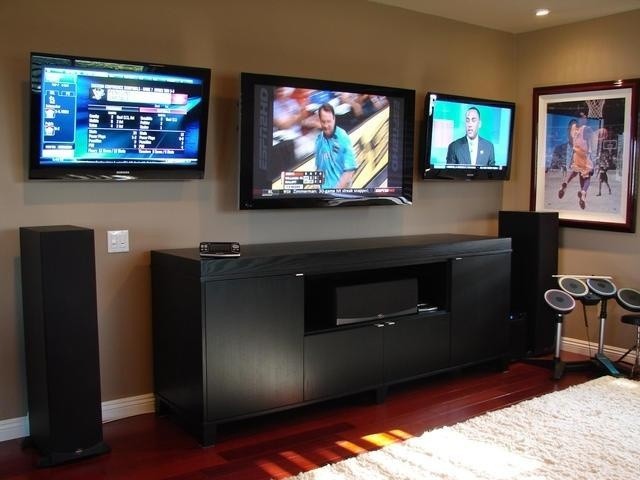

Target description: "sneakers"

left=578, top=190, right=586, bottom=210
left=557, top=182, right=567, bottom=198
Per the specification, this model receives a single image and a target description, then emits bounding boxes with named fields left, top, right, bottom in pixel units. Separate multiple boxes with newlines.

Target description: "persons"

left=313, top=104, right=357, bottom=188
left=272, top=86, right=390, bottom=147
left=551, top=119, right=612, bottom=209
left=446, top=107, right=495, bottom=164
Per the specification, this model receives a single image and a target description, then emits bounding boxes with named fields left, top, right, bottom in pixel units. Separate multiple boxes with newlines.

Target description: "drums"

left=545, top=289, right=575, bottom=314
left=616, top=288, right=640, bottom=312
left=587, top=279, right=617, bottom=299
left=559, top=276, right=589, bottom=300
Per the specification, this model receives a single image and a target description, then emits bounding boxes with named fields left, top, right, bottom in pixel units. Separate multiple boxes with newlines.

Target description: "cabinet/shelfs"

left=149, top=231, right=514, bottom=444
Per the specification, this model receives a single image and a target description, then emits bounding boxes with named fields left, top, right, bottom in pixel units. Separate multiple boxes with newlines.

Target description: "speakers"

left=499, top=210, right=559, bottom=357
left=20, top=225, right=105, bottom=454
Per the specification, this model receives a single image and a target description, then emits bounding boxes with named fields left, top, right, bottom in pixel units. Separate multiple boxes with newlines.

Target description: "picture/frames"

left=530, top=76, right=639, bottom=235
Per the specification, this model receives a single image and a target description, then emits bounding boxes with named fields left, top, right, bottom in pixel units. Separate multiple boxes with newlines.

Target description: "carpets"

left=283, top=373, right=640, bottom=480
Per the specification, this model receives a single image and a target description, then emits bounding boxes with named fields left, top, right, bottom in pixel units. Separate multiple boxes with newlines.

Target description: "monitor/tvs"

left=29, top=51, right=211, bottom=180
left=420, top=91, right=516, bottom=181
left=241, top=71, right=415, bottom=209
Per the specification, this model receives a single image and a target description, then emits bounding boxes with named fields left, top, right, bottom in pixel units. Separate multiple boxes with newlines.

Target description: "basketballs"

left=597, top=128, right=608, bottom=140
